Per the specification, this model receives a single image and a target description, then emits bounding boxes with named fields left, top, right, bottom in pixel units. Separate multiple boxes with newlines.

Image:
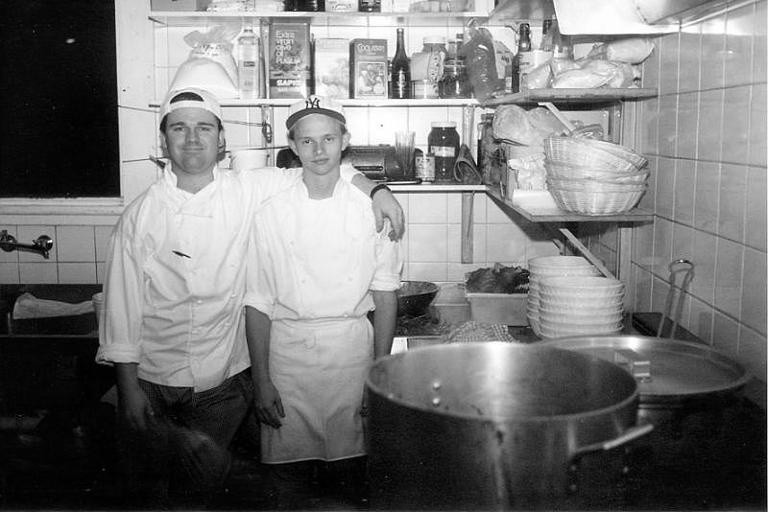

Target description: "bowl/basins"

left=525, top=254, right=625, bottom=339
left=396, top=279, right=440, bottom=316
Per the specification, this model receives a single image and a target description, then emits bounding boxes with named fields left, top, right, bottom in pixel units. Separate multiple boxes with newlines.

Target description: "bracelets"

left=368, top=183, right=391, bottom=199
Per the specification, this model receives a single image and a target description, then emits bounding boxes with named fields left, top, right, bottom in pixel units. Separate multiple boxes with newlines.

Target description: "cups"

left=395, top=130, right=417, bottom=181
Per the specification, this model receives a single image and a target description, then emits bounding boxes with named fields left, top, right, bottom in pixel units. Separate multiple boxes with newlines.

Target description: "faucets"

left=33, top=237, right=49, bottom=259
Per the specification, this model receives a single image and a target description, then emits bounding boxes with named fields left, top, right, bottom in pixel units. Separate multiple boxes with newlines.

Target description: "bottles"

left=237, top=23, right=262, bottom=99
left=389, top=27, right=412, bottom=98
left=511, top=22, right=532, bottom=93
left=444, top=31, right=465, bottom=77
left=427, top=121, right=460, bottom=180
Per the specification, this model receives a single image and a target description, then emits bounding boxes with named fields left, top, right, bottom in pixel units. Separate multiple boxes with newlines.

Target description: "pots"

left=532, top=259, right=753, bottom=467
left=357, top=342, right=658, bottom=511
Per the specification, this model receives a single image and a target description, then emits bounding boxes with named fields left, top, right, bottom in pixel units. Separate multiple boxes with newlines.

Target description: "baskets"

left=542, top=135, right=648, bottom=216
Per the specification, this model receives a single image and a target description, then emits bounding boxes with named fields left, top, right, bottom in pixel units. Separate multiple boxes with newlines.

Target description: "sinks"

left=8, top=290, right=100, bottom=338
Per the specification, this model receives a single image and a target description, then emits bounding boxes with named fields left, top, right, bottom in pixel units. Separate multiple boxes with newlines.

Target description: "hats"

left=285, top=97, right=346, bottom=130
left=161, top=88, right=226, bottom=161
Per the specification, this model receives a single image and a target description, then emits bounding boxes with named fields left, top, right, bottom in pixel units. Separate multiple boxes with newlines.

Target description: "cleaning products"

left=237, top=28, right=260, bottom=100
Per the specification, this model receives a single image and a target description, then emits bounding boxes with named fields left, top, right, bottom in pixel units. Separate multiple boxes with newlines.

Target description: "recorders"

left=276, top=145, right=424, bottom=185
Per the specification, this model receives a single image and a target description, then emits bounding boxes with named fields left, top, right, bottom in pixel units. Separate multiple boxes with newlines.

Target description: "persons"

left=96, top=84, right=405, bottom=511
left=241, top=96, right=402, bottom=511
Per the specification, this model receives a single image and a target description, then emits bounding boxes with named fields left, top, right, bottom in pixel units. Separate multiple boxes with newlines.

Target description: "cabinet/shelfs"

left=142, top=0, right=682, bottom=222
